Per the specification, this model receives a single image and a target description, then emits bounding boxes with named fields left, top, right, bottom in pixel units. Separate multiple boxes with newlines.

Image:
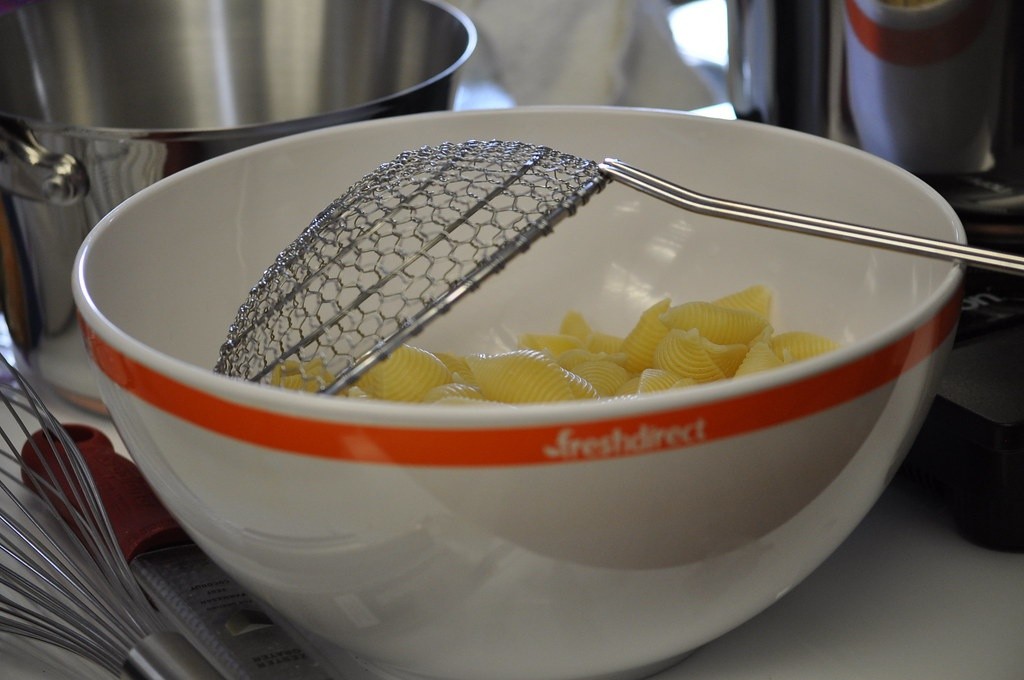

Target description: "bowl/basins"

left=71, top=106, right=967, bottom=680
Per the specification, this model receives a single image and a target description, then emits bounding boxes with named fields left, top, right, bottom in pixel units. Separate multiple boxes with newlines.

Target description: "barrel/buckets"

left=1, top=1, right=477, bottom=422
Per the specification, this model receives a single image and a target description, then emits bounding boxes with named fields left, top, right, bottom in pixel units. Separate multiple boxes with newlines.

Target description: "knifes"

left=21, top=423, right=338, bottom=679
